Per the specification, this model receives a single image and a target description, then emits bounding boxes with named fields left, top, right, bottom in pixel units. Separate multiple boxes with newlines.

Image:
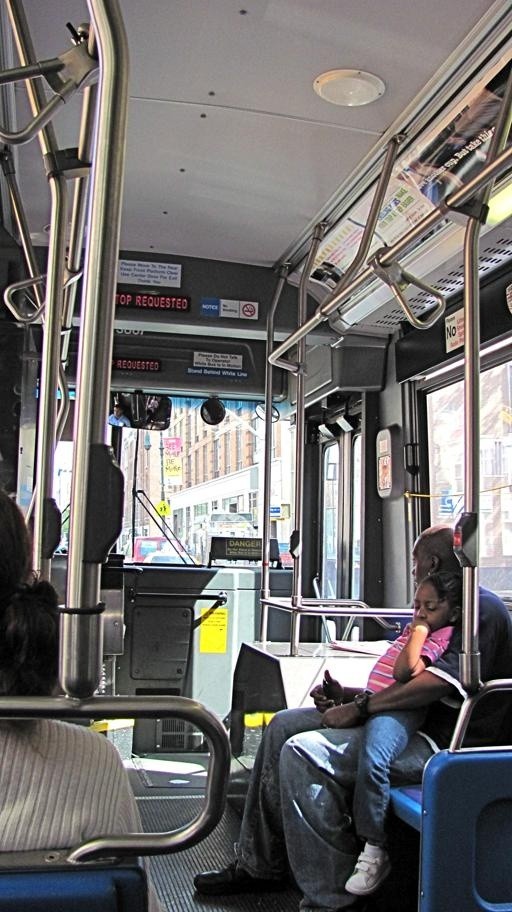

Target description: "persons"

left=344, top=570, right=463, bottom=895
left=1, top=576, right=161, bottom=910
left=193, top=522, right=510, bottom=912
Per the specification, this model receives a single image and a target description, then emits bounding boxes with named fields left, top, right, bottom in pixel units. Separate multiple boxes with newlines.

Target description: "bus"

left=133, top=537, right=180, bottom=563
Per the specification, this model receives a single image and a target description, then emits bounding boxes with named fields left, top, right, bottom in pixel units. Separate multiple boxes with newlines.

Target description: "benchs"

left=390, top=782, right=422, bottom=831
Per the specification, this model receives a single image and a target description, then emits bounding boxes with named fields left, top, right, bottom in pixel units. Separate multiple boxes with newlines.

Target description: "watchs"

left=353, top=692, right=369, bottom=725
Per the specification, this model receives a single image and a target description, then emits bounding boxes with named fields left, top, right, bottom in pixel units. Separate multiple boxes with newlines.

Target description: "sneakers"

left=345, top=851, right=391, bottom=895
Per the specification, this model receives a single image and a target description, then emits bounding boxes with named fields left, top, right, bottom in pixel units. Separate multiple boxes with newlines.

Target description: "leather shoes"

left=194, top=860, right=290, bottom=894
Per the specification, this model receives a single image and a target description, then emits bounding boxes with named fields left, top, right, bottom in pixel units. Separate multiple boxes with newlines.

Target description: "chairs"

left=0, top=867, right=148, bottom=911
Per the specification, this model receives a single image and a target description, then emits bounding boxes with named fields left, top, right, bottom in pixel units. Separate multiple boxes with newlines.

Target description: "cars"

left=143, top=554, right=197, bottom=564
